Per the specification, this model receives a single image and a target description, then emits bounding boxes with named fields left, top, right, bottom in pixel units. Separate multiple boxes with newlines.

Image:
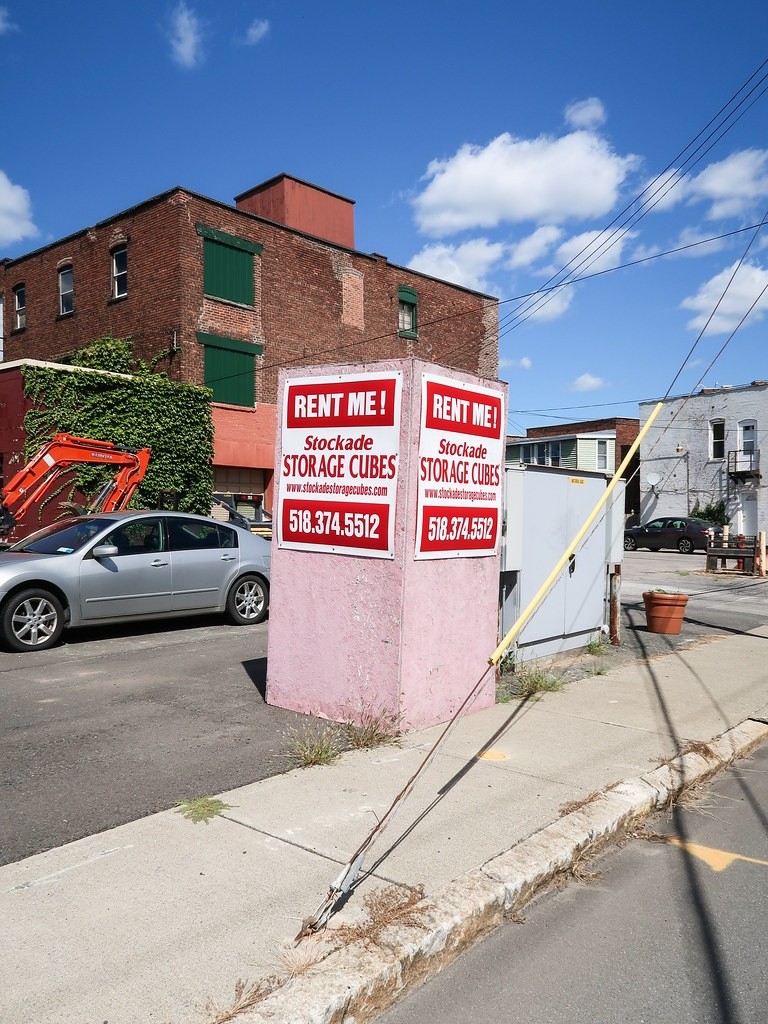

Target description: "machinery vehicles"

left=0, top=432, right=150, bottom=552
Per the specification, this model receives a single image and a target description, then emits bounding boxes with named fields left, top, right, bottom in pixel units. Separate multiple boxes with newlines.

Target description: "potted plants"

left=642, top=588, right=689, bottom=635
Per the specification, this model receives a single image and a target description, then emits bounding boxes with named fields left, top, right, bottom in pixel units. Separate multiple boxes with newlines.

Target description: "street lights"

left=676, top=443, right=690, bottom=517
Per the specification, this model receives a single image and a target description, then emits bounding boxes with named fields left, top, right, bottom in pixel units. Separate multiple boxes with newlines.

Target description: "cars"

left=0, top=510, right=271, bottom=653
left=624, top=517, right=732, bottom=554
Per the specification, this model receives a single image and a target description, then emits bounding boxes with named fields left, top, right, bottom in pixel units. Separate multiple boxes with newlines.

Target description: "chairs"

left=680, top=523, right=685, bottom=528
left=206, top=532, right=226, bottom=549
left=109, top=534, right=129, bottom=548
left=668, top=522, right=675, bottom=528
left=144, top=535, right=158, bottom=551
